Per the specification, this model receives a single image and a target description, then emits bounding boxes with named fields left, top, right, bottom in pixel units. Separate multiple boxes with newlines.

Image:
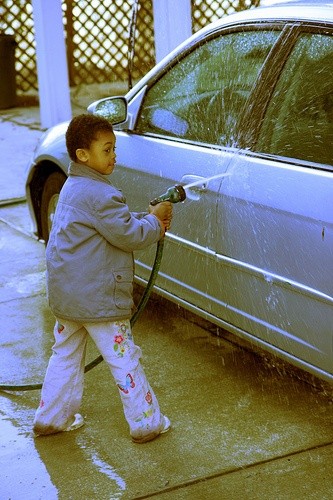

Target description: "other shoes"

left=130, top=417, right=171, bottom=443
left=63, top=414, right=84, bottom=432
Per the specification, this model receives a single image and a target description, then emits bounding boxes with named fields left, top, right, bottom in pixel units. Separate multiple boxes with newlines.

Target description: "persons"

left=32, top=113, right=172, bottom=443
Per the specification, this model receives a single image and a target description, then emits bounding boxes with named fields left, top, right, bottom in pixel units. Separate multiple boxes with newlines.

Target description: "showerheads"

left=150, top=185, right=186, bottom=206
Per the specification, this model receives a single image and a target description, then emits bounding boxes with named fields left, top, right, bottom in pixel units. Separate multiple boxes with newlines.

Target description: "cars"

left=24, top=0, right=333, bottom=394
